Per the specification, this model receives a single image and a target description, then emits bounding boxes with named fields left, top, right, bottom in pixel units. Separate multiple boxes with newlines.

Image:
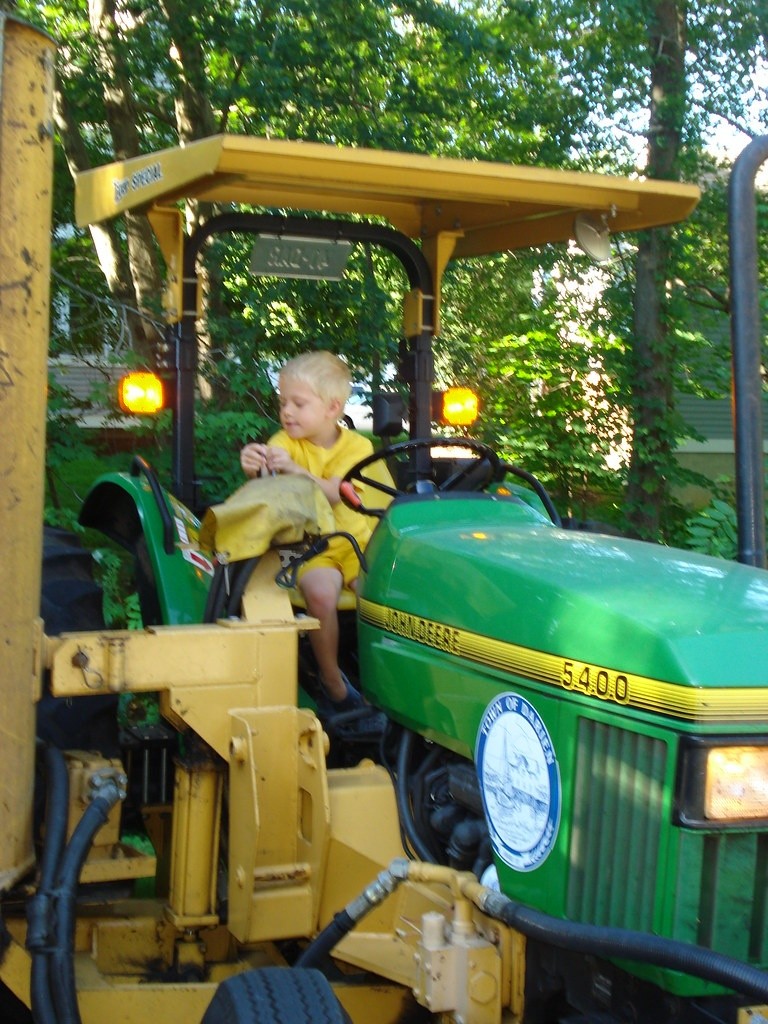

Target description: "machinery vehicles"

left=1, top=0, right=768, bottom=1023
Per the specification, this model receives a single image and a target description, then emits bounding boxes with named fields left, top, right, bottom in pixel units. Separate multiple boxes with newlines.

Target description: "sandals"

left=318, top=667, right=363, bottom=710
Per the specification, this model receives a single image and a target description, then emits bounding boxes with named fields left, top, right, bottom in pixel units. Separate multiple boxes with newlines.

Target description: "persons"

left=240, top=350, right=398, bottom=714
